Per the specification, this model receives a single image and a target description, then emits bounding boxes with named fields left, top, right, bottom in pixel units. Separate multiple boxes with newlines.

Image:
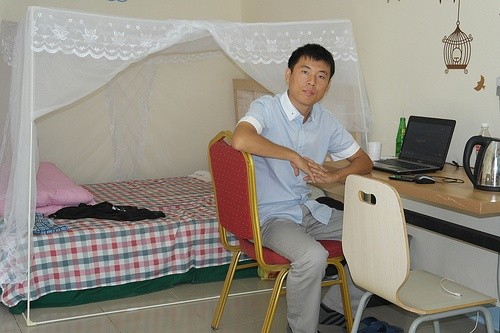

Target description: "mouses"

left=416, top=176, right=436, bottom=183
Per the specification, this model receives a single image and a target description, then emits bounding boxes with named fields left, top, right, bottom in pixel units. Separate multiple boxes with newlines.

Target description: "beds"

left=0, top=79, right=365, bottom=314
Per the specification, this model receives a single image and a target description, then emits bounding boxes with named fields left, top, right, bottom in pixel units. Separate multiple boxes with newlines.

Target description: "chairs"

left=342, top=174, right=497, bottom=333
left=208, top=130, right=352, bottom=333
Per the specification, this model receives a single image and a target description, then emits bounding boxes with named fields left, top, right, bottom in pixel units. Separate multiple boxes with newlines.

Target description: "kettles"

left=463, top=135, right=500, bottom=192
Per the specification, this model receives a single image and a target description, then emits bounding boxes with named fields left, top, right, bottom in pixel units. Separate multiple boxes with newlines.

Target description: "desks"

left=302, top=160, right=500, bottom=333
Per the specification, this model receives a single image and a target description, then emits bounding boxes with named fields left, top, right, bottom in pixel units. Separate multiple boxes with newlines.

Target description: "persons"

left=231, top=43, right=374, bottom=333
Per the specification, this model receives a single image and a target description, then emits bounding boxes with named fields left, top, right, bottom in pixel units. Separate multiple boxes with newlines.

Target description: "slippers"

left=343, top=316, right=405, bottom=333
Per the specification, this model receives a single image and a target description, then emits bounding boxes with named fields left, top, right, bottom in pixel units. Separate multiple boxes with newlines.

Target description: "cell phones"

left=389, top=175, right=415, bottom=181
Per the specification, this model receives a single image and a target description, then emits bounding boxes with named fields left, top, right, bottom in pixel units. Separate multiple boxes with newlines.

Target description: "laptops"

left=371, top=116, right=456, bottom=173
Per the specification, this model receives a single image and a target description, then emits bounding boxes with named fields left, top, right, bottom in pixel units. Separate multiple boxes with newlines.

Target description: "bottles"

left=395, top=117, right=407, bottom=157
left=475, top=123, right=493, bottom=156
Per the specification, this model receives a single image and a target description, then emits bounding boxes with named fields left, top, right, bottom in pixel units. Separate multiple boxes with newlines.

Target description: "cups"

left=368, top=141, right=382, bottom=162
